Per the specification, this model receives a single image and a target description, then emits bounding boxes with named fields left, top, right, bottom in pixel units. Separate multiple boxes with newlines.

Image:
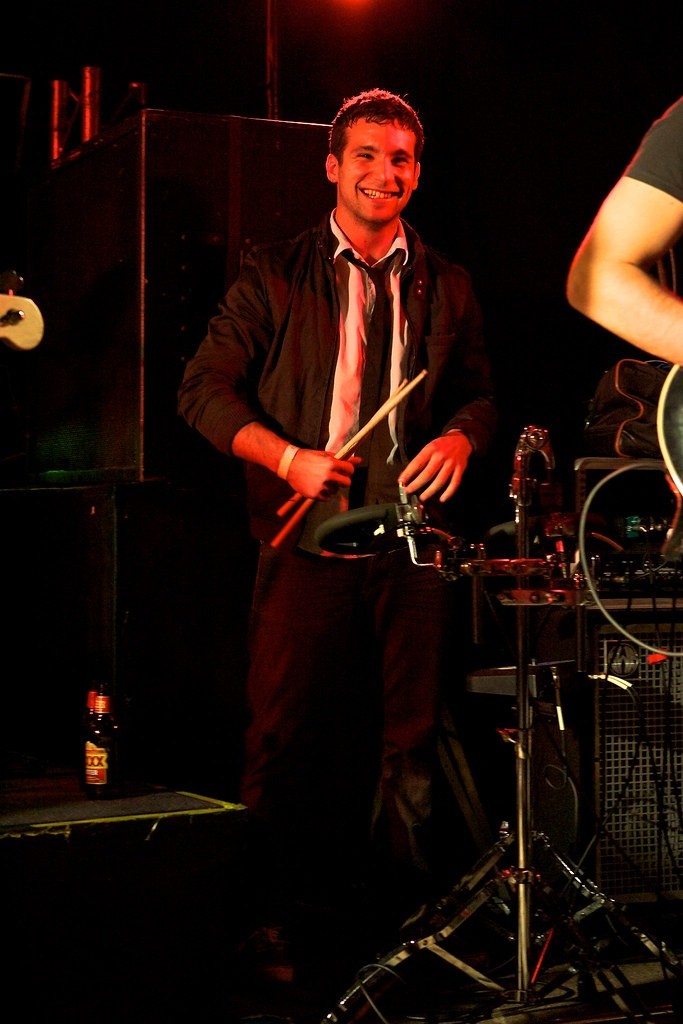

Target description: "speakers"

left=0, top=479, right=261, bottom=787
left=27, top=105, right=336, bottom=481
left=529, top=618, right=683, bottom=906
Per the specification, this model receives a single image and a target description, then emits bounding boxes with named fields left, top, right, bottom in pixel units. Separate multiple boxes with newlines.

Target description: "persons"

left=565, top=88, right=683, bottom=370
left=174, top=87, right=512, bottom=982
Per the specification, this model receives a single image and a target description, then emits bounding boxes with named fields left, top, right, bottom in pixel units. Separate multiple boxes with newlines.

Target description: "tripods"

left=324, top=427, right=683, bottom=1024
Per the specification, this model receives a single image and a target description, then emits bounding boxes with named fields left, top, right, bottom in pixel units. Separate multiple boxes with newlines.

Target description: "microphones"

left=521, top=449, right=550, bottom=517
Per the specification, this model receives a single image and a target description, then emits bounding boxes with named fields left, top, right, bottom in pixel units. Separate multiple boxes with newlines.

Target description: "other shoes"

left=237, top=925, right=293, bottom=982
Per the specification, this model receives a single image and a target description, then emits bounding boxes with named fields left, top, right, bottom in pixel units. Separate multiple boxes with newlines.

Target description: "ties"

left=340, top=248, right=399, bottom=510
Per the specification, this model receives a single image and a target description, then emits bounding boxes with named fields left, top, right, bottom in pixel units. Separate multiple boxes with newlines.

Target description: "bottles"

left=85, top=682, right=120, bottom=800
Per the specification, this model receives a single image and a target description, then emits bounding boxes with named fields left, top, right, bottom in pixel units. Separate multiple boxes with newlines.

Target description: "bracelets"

left=277, top=444, right=300, bottom=481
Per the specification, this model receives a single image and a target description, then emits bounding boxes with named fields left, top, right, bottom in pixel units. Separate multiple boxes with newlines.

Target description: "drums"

left=314, top=503, right=426, bottom=557
